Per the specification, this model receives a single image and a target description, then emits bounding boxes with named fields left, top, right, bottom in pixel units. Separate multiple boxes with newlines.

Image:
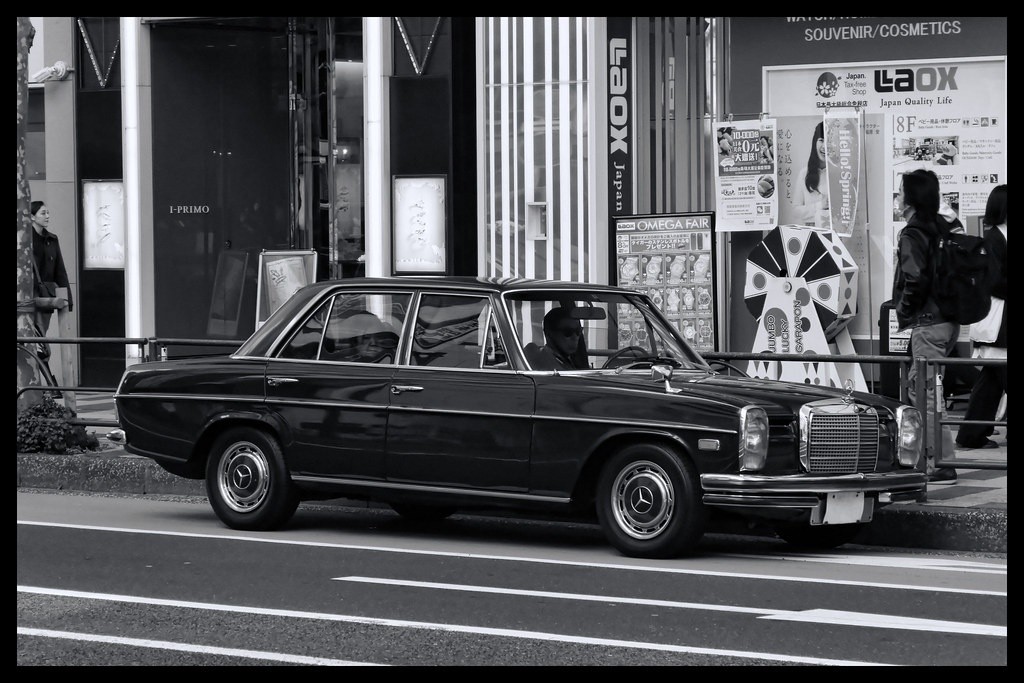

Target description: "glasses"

left=549, top=325, right=584, bottom=338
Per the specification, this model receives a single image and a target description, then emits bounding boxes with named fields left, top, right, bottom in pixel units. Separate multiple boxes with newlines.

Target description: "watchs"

left=619, top=254, right=714, bottom=352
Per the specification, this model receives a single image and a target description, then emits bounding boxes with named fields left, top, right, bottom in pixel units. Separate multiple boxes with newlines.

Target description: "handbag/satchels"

left=38, top=282, right=60, bottom=299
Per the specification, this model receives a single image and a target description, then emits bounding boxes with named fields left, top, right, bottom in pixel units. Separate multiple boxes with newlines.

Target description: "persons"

left=792, top=122, right=828, bottom=226
left=889, top=169, right=1008, bottom=500
left=31, top=201, right=73, bottom=398
left=543, top=308, right=582, bottom=371
left=343, top=310, right=383, bottom=364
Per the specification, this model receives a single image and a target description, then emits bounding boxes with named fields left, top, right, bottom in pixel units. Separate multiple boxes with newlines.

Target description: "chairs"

left=339, top=310, right=386, bottom=348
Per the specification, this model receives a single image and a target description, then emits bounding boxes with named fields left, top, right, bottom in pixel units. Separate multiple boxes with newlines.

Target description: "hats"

left=904, top=169, right=940, bottom=198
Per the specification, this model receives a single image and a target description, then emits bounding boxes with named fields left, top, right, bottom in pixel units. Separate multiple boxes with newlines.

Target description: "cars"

left=112, top=274, right=928, bottom=559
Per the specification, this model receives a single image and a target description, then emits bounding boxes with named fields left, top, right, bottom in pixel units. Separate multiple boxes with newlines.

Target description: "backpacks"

left=903, top=219, right=992, bottom=325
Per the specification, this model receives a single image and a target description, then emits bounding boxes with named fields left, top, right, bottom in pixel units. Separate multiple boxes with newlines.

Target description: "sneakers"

left=929, top=467, right=957, bottom=479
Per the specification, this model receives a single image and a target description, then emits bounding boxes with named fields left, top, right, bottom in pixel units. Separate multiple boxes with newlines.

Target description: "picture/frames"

left=80, top=178, right=124, bottom=271
left=610, top=209, right=718, bottom=352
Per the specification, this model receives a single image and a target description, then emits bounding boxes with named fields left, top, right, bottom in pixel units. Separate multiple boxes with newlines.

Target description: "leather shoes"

left=958, top=439, right=998, bottom=451
